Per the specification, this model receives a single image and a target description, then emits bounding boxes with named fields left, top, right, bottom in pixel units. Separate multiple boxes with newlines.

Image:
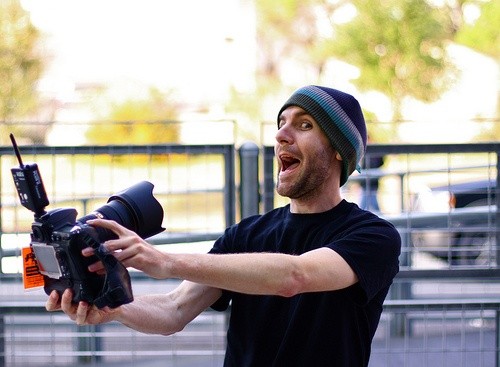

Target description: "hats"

left=278, top=84, right=367, bottom=186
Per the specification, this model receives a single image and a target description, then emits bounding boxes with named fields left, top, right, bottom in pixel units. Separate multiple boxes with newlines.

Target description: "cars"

left=404, top=178, right=499, bottom=267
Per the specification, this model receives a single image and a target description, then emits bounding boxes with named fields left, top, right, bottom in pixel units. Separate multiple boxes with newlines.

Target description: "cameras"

left=7, top=134, right=166, bottom=308
